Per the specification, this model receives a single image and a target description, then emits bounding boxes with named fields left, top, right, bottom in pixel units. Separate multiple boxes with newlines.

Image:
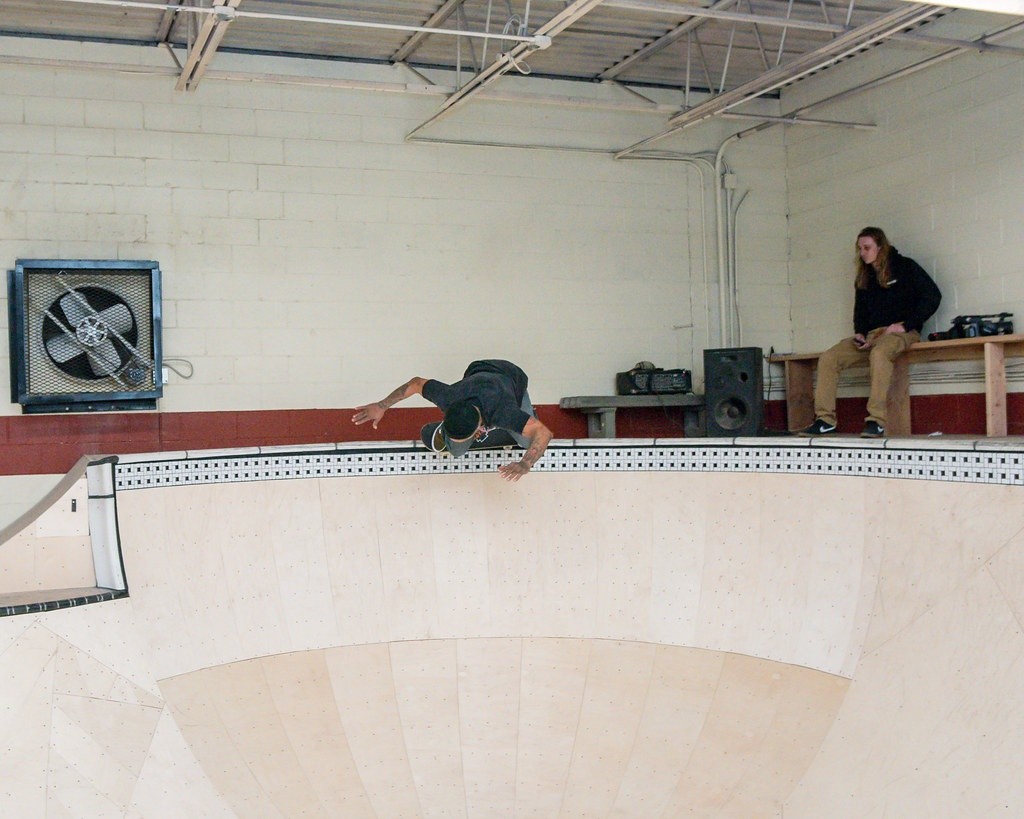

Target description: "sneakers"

left=860, top=421, right=884, bottom=437
left=799, top=419, right=837, bottom=437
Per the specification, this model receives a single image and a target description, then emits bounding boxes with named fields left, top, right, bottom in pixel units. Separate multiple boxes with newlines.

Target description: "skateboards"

left=420, top=410, right=539, bottom=452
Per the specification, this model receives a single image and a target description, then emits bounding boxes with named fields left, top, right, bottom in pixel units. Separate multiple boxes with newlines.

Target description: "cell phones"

left=853, top=338, right=865, bottom=346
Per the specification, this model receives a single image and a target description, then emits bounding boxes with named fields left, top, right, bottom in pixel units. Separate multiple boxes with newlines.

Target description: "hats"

left=443, top=405, right=481, bottom=457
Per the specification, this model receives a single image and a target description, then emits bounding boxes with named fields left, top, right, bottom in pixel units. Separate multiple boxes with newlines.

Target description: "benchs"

left=765, top=332, right=1024, bottom=437
left=560, top=395, right=710, bottom=439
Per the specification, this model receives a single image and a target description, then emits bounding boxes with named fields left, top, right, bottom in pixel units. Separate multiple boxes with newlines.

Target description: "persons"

left=799, top=227, right=942, bottom=440
left=352, top=359, right=553, bottom=483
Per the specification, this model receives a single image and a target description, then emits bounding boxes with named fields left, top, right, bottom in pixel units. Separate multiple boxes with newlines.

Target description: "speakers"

left=703, top=347, right=765, bottom=438
